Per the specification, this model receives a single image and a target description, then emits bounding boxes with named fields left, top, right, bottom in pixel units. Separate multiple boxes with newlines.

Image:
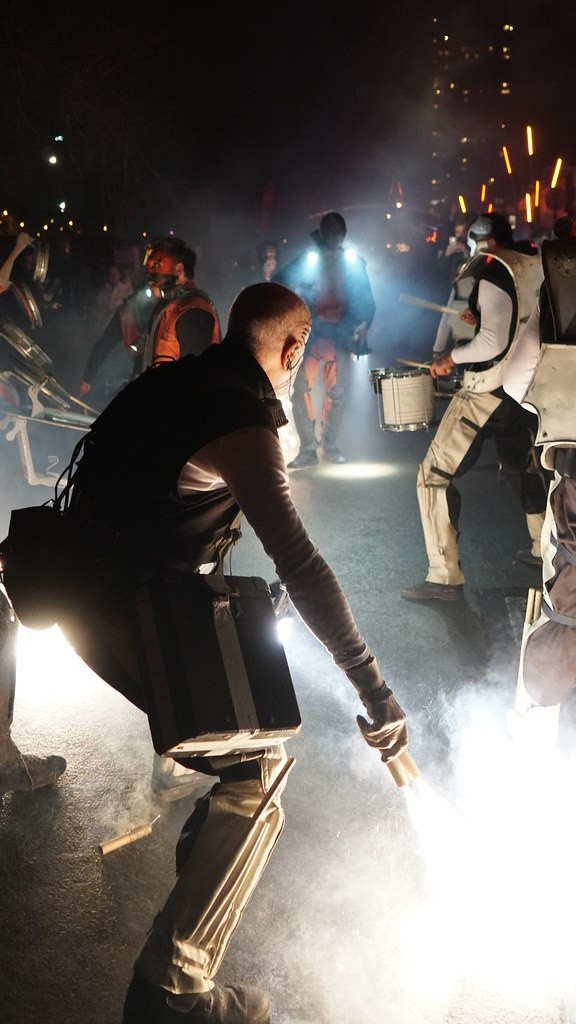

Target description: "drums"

left=0, top=237, right=72, bottom=421
left=369, top=363, right=464, bottom=433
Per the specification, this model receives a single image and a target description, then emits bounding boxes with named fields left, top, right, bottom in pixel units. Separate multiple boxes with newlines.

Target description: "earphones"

left=286, top=355, right=293, bottom=370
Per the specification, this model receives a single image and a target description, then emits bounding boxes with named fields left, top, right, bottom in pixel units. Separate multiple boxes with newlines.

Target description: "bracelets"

left=359, top=682, right=393, bottom=706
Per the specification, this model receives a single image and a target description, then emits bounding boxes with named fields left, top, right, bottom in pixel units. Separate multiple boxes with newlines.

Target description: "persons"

left=270, top=209, right=376, bottom=468
left=402, top=211, right=576, bottom=646
left=0, top=206, right=404, bottom=1024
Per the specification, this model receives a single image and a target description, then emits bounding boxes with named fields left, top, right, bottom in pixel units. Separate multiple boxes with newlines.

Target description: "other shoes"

left=286, top=449, right=319, bottom=469
left=0, top=754, right=67, bottom=796
left=151, top=765, right=220, bottom=801
left=400, top=581, right=464, bottom=603
left=517, top=549, right=543, bottom=567
left=123, top=974, right=272, bottom=1024
left=322, top=448, right=347, bottom=464
